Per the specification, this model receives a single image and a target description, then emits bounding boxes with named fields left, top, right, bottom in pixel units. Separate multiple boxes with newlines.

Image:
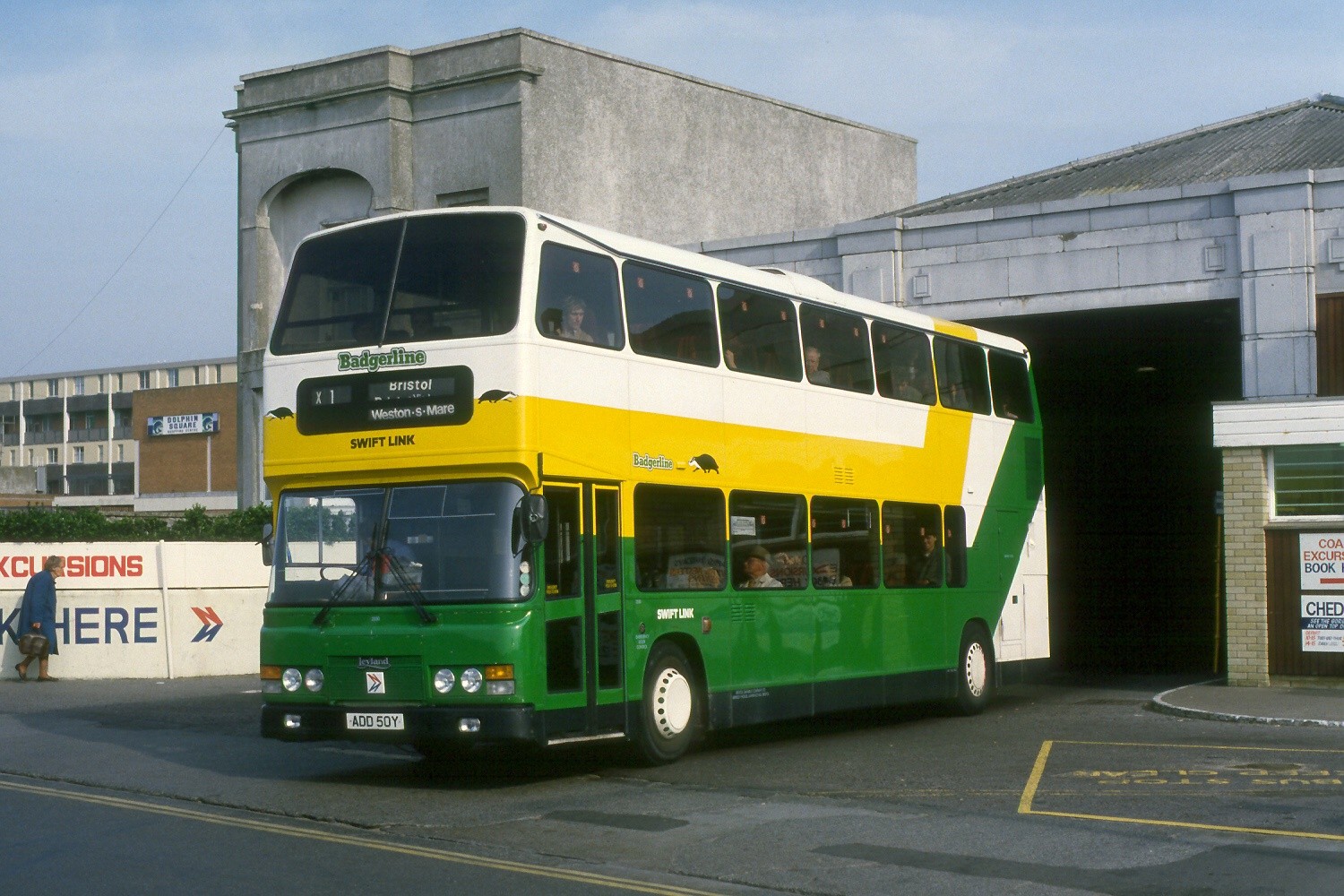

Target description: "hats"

left=744, top=545, right=774, bottom=566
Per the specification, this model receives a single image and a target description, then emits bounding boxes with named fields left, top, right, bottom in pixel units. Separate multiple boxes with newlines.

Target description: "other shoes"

left=15, top=663, right=29, bottom=681
left=37, top=676, right=59, bottom=682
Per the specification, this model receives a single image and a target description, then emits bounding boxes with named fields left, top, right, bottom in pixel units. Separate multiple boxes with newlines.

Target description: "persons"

left=881, top=374, right=922, bottom=403
left=688, top=567, right=719, bottom=589
left=739, top=546, right=783, bottom=588
left=805, top=346, right=830, bottom=386
left=350, top=316, right=377, bottom=343
left=554, top=296, right=594, bottom=343
left=410, top=308, right=439, bottom=339
left=914, top=528, right=952, bottom=585
left=357, top=522, right=415, bottom=588
left=815, top=560, right=852, bottom=587
left=16, top=556, right=63, bottom=682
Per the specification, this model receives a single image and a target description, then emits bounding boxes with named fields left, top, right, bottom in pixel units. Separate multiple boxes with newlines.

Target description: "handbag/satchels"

left=19, top=625, right=50, bottom=657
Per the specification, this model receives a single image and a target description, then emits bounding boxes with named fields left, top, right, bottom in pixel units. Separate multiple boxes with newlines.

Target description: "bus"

left=260, top=202, right=1052, bottom=766
